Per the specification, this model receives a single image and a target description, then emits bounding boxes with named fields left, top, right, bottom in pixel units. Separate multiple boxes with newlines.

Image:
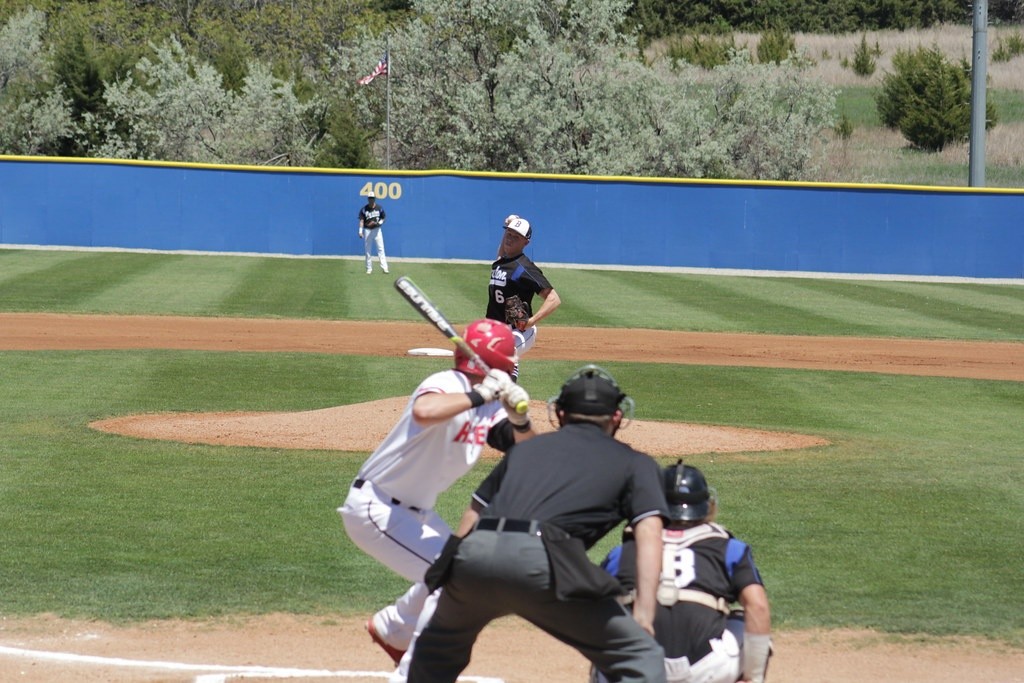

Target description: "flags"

left=358, top=51, right=389, bottom=84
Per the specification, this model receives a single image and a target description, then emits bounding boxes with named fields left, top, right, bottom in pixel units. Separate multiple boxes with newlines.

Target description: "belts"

left=687, top=644, right=711, bottom=667
left=354, top=478, right=418, bottom=511
left=478, top=518, right=531, bottom=533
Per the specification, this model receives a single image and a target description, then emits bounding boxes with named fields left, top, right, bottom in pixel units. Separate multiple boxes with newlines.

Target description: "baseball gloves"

left=503, top=294, right=529, bottom=332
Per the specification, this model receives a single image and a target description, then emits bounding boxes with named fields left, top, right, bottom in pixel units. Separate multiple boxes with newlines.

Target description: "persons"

left=409, top=363, right=672, bottom=683
left=485, top=214, right=561, bottom=384
left=358, top=191, right=389, bottom=274
left=587, top=461, right=772, bottom=683
left=338, top=319, right=538, bottom=683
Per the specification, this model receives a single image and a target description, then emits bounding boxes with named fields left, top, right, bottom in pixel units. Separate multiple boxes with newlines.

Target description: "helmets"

left=454, top=317, right=516, bottom=376
left=660, top=464, right=708, bottom=522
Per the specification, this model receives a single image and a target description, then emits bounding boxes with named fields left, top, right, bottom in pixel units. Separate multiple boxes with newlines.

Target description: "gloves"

left=498, top=379, right=530, bottom=427
left=466, top=369, right=512, bottom=408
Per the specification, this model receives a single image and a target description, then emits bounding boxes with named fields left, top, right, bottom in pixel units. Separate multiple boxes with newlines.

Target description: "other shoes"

left=363, top=617, right=407, bottom=665
left=384, top=269, right=390, bottom=274
left=366, top=267, right=372, bottom=275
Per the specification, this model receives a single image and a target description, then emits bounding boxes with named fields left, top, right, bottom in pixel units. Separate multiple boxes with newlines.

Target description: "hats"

left=367, top=192, right=375, bottom=197
left=502, top=218, right=531, bottom=240
left=558, top=374, right=620, bottom=416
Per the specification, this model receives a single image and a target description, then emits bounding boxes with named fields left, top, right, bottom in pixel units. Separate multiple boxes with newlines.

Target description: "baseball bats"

left=393, top=274, right=528, bottom=415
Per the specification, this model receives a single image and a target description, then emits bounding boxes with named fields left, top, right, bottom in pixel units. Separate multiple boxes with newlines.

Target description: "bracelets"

left=511, top=418, right=531, bottom=433
left=465, top=392, right=485, bottom=408
